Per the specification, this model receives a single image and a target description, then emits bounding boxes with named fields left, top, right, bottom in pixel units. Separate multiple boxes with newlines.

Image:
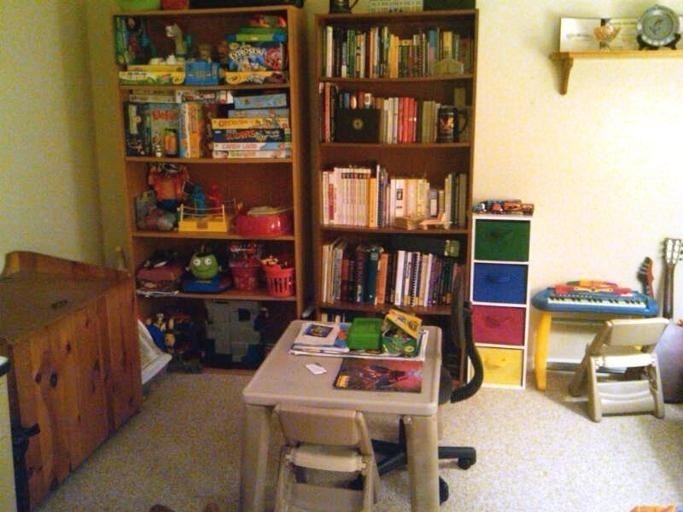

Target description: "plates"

left=637, top=7, right=679, bottom=47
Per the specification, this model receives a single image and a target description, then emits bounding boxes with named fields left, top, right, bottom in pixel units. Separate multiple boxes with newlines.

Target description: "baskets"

left=263, top=255, right=295, bottom=297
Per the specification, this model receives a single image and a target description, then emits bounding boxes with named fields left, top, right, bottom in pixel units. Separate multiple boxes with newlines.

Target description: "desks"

left=238, top=319, right=445, bottom=511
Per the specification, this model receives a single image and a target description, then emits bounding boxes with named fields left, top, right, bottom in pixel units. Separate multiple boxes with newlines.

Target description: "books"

left=295, top=317, right=351, bottom=354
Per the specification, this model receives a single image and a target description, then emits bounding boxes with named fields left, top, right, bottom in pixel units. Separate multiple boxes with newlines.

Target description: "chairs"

left=351, top=274, right=485, bottom=505
left=267, top=403, right=388, bottom=510
left=568, top=317, right=669, bottom=423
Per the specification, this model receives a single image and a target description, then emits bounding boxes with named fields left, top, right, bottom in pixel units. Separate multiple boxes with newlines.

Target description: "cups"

left=437, top=108, right=466, bottom=143
left=163, top=134, right=178, bottom=157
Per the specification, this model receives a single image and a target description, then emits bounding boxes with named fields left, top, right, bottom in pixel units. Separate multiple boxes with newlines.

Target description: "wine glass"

left=592, top=24, right=622, bottom=50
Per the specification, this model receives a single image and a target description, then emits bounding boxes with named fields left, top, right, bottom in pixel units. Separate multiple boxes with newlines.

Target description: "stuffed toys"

left=184, top=241, right=227, bottom=285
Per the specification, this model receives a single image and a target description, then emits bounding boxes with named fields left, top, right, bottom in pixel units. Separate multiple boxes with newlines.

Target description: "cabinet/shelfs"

left=107, top=5, right=312, bottom=373
left=470, top=211, right=535, bottom=388
left=310, top=7, right=482, bottom=391
left=0, top=248, right=146, bottom=512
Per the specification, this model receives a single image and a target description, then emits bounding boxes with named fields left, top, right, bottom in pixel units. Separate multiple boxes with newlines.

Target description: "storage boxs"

left=204, top=300, right=262, bottom=367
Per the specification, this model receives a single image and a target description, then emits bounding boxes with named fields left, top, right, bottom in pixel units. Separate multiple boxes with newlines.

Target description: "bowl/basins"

left=231, top=215, right=288, bottom=237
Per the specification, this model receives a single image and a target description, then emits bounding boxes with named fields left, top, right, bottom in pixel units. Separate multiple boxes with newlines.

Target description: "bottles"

left=154, top=146, right=162, bottom=158
